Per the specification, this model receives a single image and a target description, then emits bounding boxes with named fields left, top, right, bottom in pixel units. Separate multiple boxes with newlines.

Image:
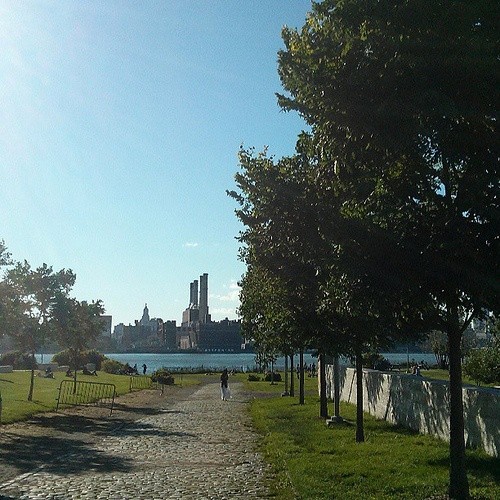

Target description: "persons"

left=141, top=363, right=147, bottom=374
left=133, top=363, right=138, bottom=371
left=36, top=365, right=74, bottom=379
left=219, top=369, right=231, bottom=401
left=292, top=359, right=321, bottom=383
left=82, top=367, right=99, bottom=377
